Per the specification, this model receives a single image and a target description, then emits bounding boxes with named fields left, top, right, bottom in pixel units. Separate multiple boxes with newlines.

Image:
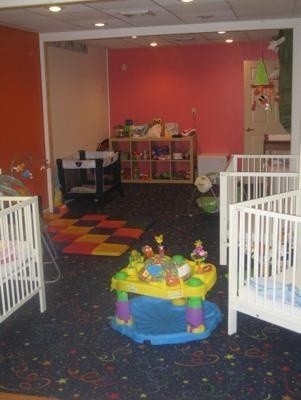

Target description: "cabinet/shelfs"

left=109, top=135, right=196, bottom=183
left=56, top=150, right=122, bottom=206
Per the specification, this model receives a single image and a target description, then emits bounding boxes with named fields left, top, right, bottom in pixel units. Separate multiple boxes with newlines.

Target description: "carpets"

left=44, top=213, right=152, bottom=256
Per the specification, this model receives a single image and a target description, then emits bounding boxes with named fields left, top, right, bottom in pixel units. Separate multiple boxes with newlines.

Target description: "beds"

left=227, top=190, right=301, bottom=336
left=1, top=195, right=47, bottom=325
left=219, top=154, right=299, bottom=265
